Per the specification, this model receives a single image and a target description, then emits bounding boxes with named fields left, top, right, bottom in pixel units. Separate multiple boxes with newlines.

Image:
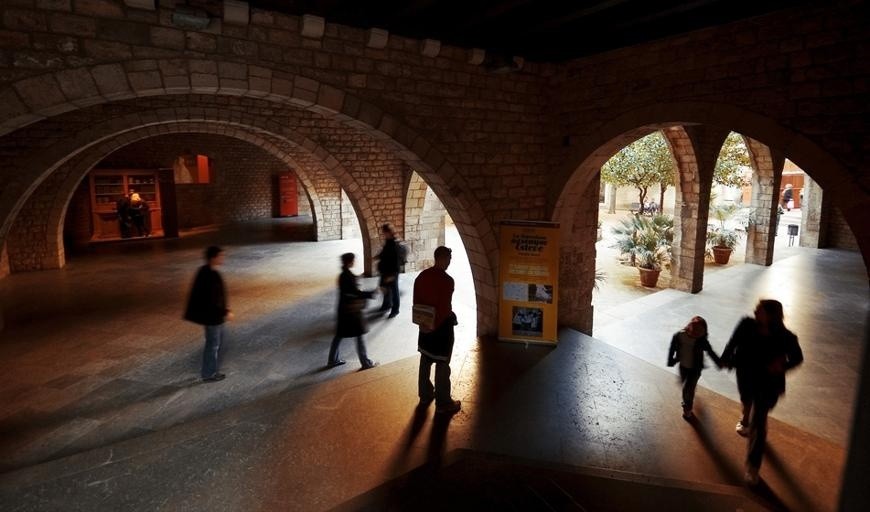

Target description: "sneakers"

left=420, top=392, right=460, bottom=413
left=736, top=422, right=750, bottom=434
left=745, top=472, right=758, bottom=485
left=328, top=359, right=375, bottom=368
left=683, top=409, right=692, bottom=418
left=204, top=374, right=225, bottom=380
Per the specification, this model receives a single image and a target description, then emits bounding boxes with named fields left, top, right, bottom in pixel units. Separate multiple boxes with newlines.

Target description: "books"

left=412, top=301, right=437, bottom=335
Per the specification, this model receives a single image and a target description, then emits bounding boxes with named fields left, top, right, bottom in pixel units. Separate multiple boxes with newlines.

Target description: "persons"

left=325, top=252, right=381, bottom=370
left=775, top=202, right=784, bottom=235
left=780, top=183, right=797, bottom=211
left=638, top=197, right=656, bottom=216
left=665, top=315, right=722, bottom=422
left=181, top=244, right=235, bottom=384
left=369, top=222, right=402, bottom=320
left=798, top=187, right=804, bottom=210
left=117, top=192, right=152, bottom=240
left=719, top=297, right=804, bottom=487
left=411, top=245, right=463, bottom=416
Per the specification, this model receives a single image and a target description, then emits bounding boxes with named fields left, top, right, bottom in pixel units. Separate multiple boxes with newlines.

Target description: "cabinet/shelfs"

left=89, top=169, right=163, bottom=238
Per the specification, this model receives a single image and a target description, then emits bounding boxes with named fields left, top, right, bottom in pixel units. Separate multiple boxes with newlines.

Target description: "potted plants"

left=629, top=233, right=672, bottom=288
left=706, top=223, right=739, bottom=264
